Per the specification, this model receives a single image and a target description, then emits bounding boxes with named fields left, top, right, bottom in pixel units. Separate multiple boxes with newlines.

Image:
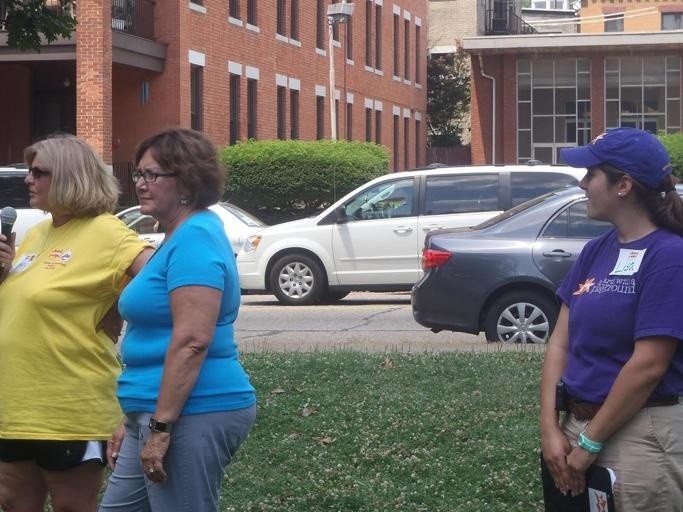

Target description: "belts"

left=564, top=395, right=679, bottom=421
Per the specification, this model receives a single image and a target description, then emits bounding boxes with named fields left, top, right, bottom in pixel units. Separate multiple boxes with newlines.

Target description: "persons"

left=0, top=132, right=156, bottom=512
left=538, top=126, right=683, bottom=511
left=95, top=125, right=258, bottom=511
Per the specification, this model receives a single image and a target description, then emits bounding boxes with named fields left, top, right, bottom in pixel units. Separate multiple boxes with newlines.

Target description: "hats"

left=560, top=127, right=674, bottom=189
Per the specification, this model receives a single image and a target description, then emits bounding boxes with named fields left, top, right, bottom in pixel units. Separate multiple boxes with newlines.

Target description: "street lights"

left=326, top=2, right=353, bottom=141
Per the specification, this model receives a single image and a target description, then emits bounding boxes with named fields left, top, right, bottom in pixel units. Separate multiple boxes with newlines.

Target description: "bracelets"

left=577, top=430, right=603, bottom=456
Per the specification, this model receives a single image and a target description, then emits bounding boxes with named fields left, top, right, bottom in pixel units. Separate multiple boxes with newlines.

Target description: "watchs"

left=147, top=415, right=172, bottom=434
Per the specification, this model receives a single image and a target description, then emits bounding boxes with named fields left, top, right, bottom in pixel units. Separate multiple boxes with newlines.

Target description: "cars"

left=410, top=184, right=683, bottom=344
left=112, top=200, right=270, bottom=296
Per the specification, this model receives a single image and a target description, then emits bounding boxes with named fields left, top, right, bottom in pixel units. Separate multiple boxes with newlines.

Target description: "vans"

left=233, top=161, right=590, bottom=307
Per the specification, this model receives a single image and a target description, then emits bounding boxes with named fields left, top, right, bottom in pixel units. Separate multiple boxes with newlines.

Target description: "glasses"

left=28, top=165, right=52, bottom=179
left=131, top=168, right=173, bottom=184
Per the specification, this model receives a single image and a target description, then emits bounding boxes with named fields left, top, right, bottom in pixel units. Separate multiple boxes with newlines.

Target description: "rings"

left=149, top=466, right=155, bottom=474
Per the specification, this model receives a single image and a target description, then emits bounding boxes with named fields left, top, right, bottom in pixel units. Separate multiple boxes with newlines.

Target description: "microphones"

left=0, top=206, right=17, bottom=270
